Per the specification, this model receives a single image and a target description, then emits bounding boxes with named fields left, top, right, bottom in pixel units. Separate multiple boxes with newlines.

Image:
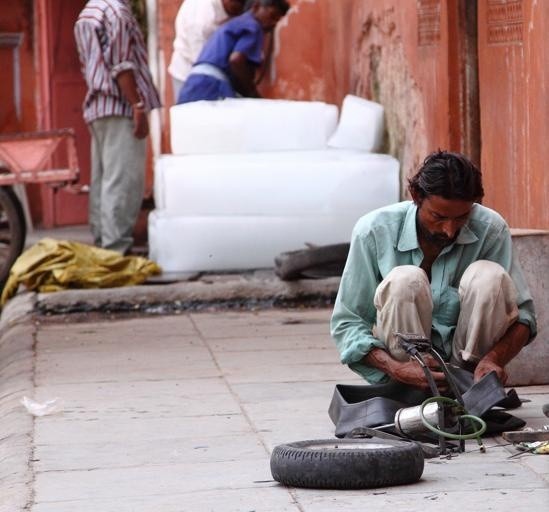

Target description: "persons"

left=331, top=148, right=537, bottom=438
left=168, top=0, right=248, bottom=104
left=176, top=0, right=290, bottom=102
left=74, top=1, right=163, bottom=258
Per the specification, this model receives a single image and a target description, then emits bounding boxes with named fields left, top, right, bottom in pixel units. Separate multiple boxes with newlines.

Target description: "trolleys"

left=0, top=127, right=80, bottom=291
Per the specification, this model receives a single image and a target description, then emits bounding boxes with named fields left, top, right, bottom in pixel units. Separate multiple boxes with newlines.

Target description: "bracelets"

left=129, top=102, right=145, bottom=109
